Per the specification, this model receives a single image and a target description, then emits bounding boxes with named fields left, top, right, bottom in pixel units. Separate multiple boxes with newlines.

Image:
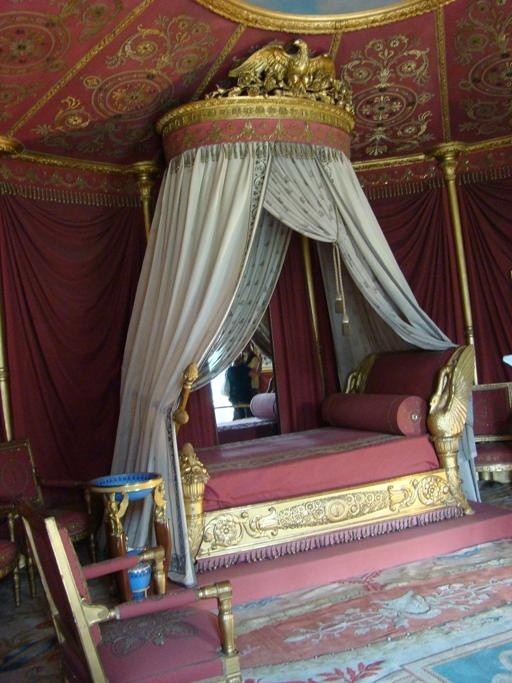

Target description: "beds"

left=120, top=344, right=475, bottom=574
left=216, top=377, right=280, bottom=444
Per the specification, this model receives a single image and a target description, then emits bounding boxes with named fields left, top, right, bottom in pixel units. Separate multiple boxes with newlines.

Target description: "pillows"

left=250, top=393, right=278, bottom=419
left=321, top=393, right=428, bottom=436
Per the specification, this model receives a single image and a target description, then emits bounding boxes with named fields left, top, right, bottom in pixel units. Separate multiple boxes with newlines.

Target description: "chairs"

left=470, top=382, right=512, bottom=481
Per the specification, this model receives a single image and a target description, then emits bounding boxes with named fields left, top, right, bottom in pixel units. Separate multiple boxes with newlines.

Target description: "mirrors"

left=206, top=281, right=278, bottom=433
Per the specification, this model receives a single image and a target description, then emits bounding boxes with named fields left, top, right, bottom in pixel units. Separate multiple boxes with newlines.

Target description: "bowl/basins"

left=85, top=471, right=162, bottom=500
